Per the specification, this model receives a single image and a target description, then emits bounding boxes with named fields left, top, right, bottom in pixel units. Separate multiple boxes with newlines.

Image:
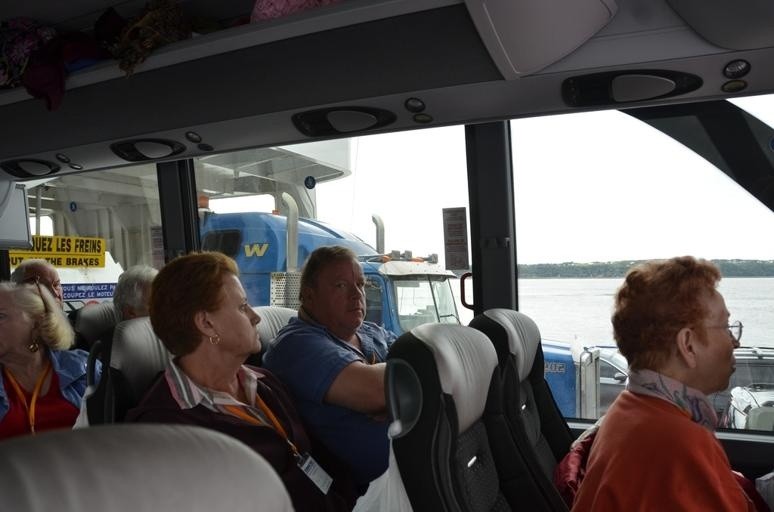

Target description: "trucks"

left=198, top=193, right=600, bottom=419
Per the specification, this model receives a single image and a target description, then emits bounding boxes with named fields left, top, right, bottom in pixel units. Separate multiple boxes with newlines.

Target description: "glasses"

left=704, top=321, right=743, bottom=342
left=17, top=272, right=47, bottom=313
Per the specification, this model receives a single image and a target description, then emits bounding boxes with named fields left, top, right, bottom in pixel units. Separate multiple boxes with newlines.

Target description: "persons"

left=261, top=244, right=399, bottom=495
left=569, top=255, right=770, bottom=512
left=0, top=263, right=161, bottom=444
left=124, top=250, right=370, bottom=512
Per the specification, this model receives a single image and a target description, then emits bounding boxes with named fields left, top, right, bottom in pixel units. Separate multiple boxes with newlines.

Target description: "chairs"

left=0, top=300, right=574, bottom=512
